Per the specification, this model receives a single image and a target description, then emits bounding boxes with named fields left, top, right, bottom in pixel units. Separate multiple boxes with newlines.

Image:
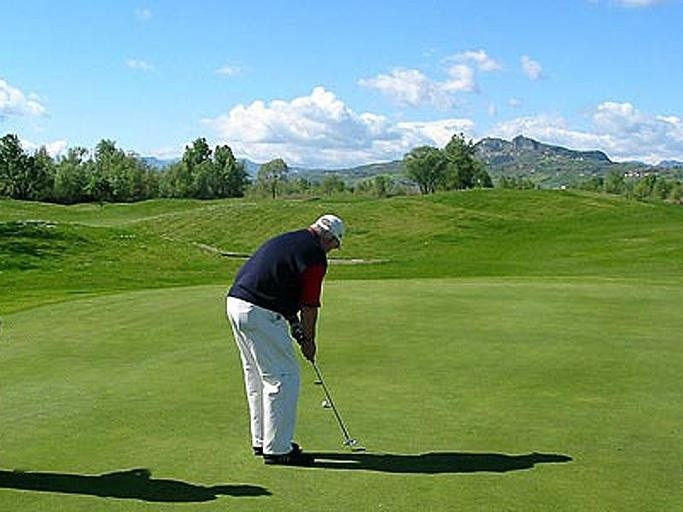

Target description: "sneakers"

left=250, top=441, right=306, bottom=467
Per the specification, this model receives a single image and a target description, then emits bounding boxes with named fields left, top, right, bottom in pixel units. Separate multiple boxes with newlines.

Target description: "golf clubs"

left=292, top=326, right=356, bottom=448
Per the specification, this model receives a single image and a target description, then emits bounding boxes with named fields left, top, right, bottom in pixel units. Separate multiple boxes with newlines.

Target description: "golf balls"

left=321, top=400, right=328, bottom=408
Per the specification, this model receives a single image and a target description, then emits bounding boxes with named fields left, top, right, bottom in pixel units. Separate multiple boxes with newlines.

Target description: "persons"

left=223, top=213, right=345, bottom=465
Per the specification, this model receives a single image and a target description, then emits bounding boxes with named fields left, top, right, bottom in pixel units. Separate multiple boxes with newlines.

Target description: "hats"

left=314, top=213, right=345, bottom=251
